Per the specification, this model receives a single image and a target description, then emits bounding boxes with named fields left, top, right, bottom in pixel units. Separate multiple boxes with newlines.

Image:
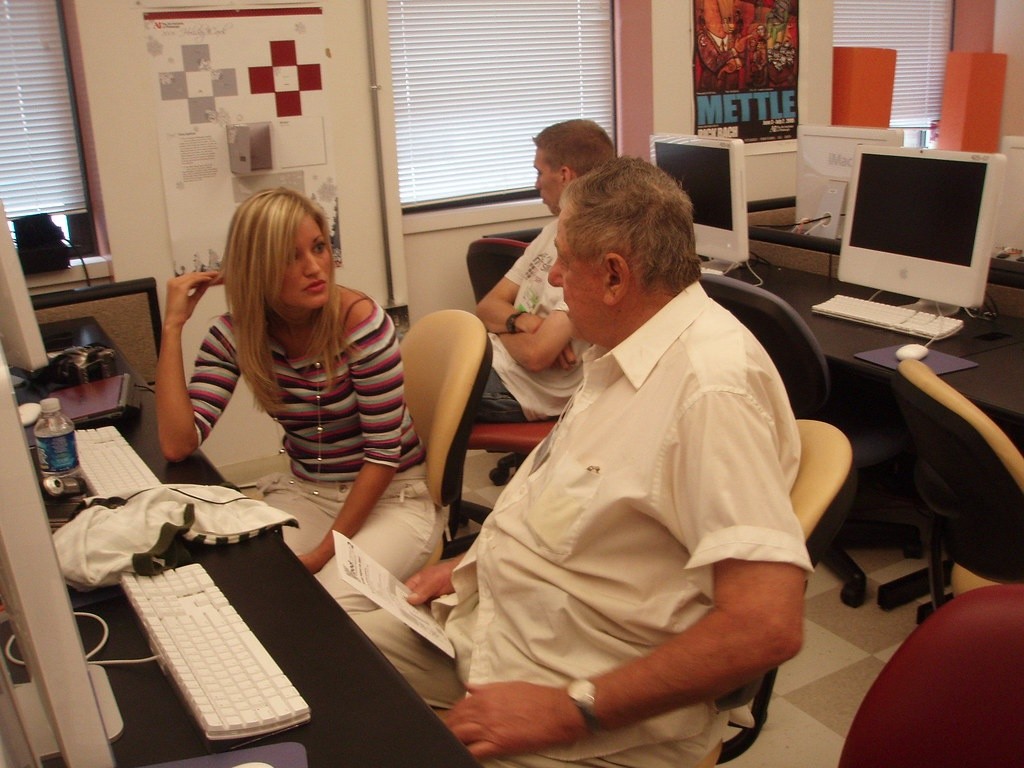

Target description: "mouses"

left=233, top=762, right=273, bottom=768
left=17, top=403, right=42, bottom=425
left=896, top=343, right=930, bottom=362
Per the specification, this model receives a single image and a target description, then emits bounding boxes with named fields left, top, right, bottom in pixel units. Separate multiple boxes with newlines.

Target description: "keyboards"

left=75, top=427, right=162, bottom=496
left=702, top=267, right=724, bottom=275
left=811, top=294, right=964, bottom=341
left=119, top=563, right=311, bottom=740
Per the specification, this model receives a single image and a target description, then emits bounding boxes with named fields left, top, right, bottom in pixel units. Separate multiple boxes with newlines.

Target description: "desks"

left=0, top=315, right=483, bottom=768
left=699, top=254, right=1024, bottom=506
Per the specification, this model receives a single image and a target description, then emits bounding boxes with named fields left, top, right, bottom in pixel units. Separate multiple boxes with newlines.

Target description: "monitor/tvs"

left=795, top=124, right=1024, bottom=316
left=650, top=133, right=751, bottom=273
left=1, top=201, right=125, bottom=768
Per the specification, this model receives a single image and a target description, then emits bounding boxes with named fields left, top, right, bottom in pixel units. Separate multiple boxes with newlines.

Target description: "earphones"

left=47, top=373, right=132, bottom=423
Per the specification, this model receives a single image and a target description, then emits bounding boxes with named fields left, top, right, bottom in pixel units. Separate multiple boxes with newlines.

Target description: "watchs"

left=506, top=311, right=528, bottom=333
left=567, top=678, right=598, bottom=732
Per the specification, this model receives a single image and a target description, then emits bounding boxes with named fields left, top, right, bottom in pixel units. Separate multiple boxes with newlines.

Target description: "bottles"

left=33, top=397, right=82, bottom=482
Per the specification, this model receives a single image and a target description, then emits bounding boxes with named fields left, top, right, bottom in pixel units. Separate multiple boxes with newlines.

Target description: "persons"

left=156, top=187, right=438, bottom=616
left=476, top=120, right=616, bottom=424
left=357, top=154, right=816, bottom=768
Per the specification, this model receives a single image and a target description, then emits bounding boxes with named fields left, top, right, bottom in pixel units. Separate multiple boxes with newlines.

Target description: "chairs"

left=398, top=310, right=493, bottom=570
left=689, top=419, right=856, bottom=768
left=891, top=360, right=1024, bottom=624
left=467, top=237, right=557, bottom=487
left=700, top=273, right=923, bottom=608
